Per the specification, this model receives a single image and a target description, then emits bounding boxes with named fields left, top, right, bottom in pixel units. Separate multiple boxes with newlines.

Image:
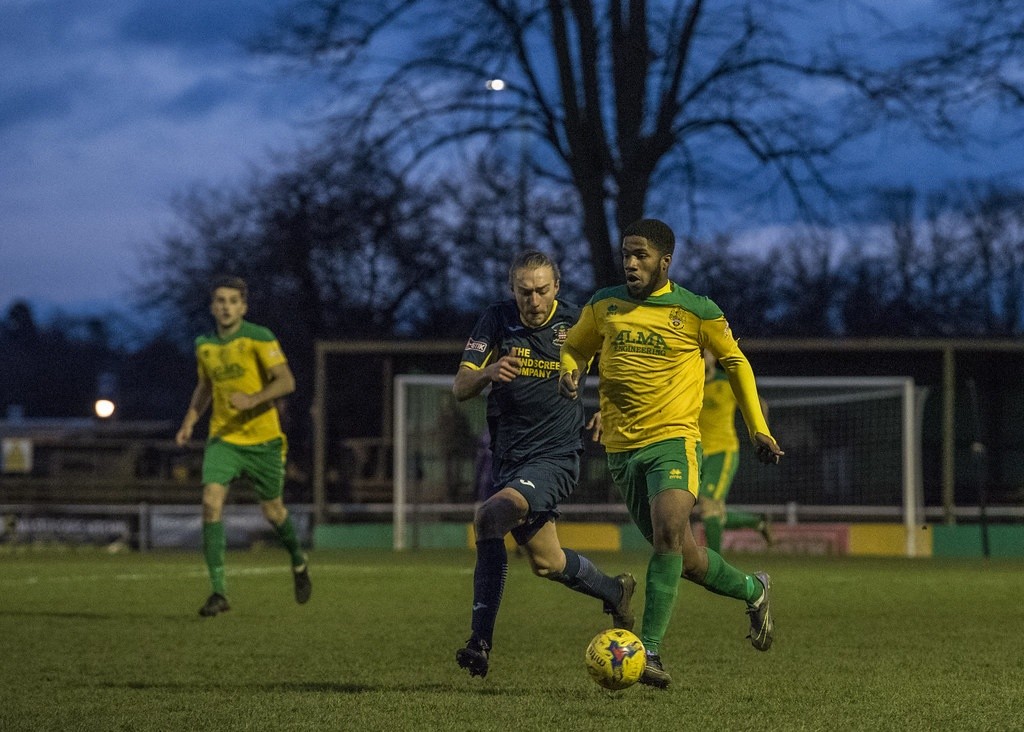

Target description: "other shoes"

left=292, top=553, right=312, bottom=605
left=199, top=593, right=231, bottom=617
left=757, top=512, right=773, bottom=545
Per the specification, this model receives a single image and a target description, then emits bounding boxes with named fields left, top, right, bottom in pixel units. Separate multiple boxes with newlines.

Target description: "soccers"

left=585, top=628, right=646, bottom=689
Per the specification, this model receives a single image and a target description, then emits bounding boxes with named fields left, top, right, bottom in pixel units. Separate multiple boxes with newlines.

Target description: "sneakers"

left=603, top=572, right=637, bottom=632
left=636, top=655, right=672, bottom=689
left=745, top=571, right=775, bottom=651
left=456, top=639, right=490, bottom=679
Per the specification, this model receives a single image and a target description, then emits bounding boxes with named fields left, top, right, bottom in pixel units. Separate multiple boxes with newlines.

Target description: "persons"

left=173, top=280, right=312, bottom=615
left=454, top=250, right=639, bottom=680
left=684, top=345, right=771, bottom=558
left=557, top=217, right=784, bottom=689
